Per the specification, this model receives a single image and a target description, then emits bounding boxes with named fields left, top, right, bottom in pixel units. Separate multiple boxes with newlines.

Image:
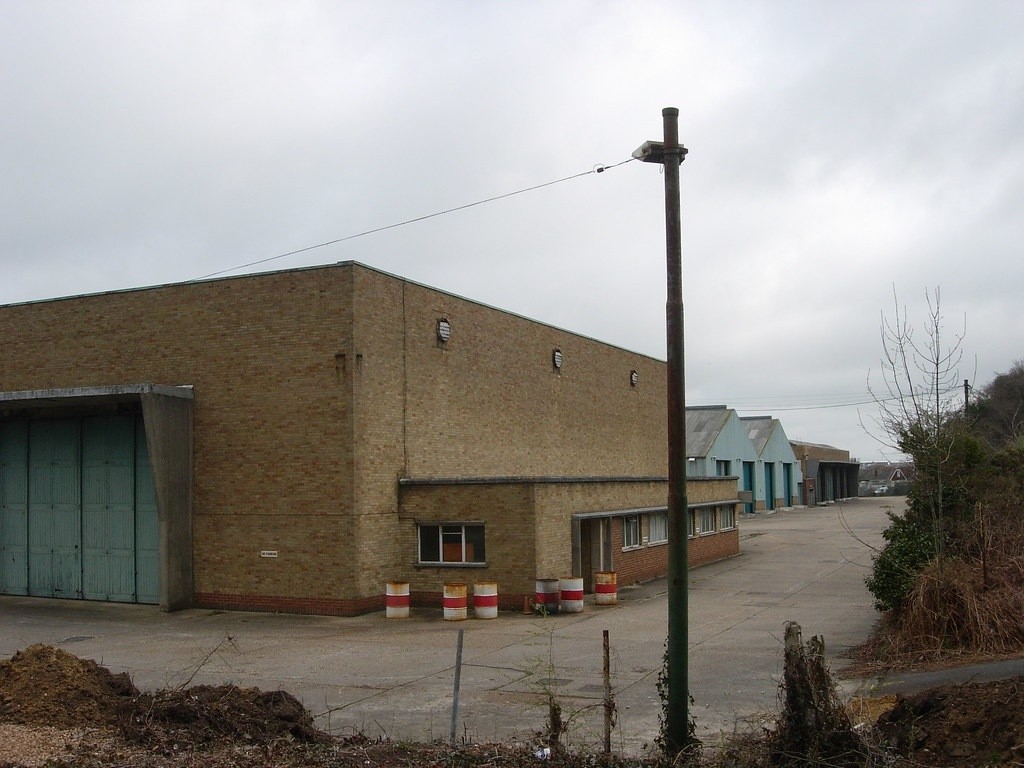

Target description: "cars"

left=873, top=486, right=888, bottom=495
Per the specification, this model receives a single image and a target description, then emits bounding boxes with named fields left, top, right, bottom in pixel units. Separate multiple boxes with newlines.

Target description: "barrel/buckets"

left=561, top=577, right=584, bottom=613
left=474, top=582, right=498, bottom=618
left=536, top=579, right=560, bottom=615
left=595, top=570, right=617, bottom=605
left=444, top=582, right=467, bottom=621
left=386, top=581, right=409, bottom=619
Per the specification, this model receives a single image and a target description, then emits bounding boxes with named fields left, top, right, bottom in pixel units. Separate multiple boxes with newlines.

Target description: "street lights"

left=633, top=107, right=689, bottom=756
